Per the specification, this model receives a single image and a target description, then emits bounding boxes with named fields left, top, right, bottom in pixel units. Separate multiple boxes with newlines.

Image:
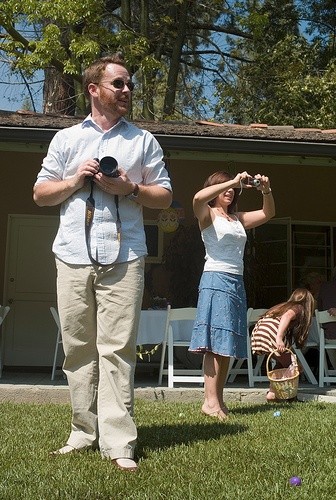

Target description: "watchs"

left=125, top=183, right=139, bottom=200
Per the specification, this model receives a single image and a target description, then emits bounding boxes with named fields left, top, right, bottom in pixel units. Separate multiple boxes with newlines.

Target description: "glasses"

left=100, top=77, right=137, bottom=91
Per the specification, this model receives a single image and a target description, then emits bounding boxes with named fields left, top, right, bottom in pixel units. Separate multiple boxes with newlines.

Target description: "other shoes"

left=46, top=442, right=91, bottom=458
left=110, top=455, right=141, bottom=476
left=266, top=390, right=279, bottom=403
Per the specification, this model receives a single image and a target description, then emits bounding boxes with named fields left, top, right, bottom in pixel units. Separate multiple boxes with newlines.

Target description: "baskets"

left=264, top=345, right=301, bottom=401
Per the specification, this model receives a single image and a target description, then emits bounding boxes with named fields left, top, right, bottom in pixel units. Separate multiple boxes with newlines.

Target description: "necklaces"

left=217, top=208, right=230, bottom=221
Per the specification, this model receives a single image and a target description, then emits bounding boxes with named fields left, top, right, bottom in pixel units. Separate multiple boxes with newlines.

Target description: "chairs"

left=136, top=305, right=336, bottom=387
left=50, top=307, right=62, bottom=380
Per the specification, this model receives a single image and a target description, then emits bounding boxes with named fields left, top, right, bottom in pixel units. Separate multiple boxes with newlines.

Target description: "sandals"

left=201, top=400, right=234, bottom=423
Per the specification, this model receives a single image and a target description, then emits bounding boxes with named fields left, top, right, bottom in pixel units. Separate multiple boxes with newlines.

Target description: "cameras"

left=247, top=177, right=261, bottom=187
left=87, top=156, right=121, bottom=183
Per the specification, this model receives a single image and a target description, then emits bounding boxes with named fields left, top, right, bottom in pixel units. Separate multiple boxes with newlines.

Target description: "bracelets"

left=262, top=188, right=271, bottom=196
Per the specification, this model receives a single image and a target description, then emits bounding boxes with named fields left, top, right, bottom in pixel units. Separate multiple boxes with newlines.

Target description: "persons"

left=188, top=170, right=275, bottom=423
left=250, top=288, right=316, bottom=402
left=33, top=52, right=172, bottom=473
left=305, top=267, right=336, bottom=370
